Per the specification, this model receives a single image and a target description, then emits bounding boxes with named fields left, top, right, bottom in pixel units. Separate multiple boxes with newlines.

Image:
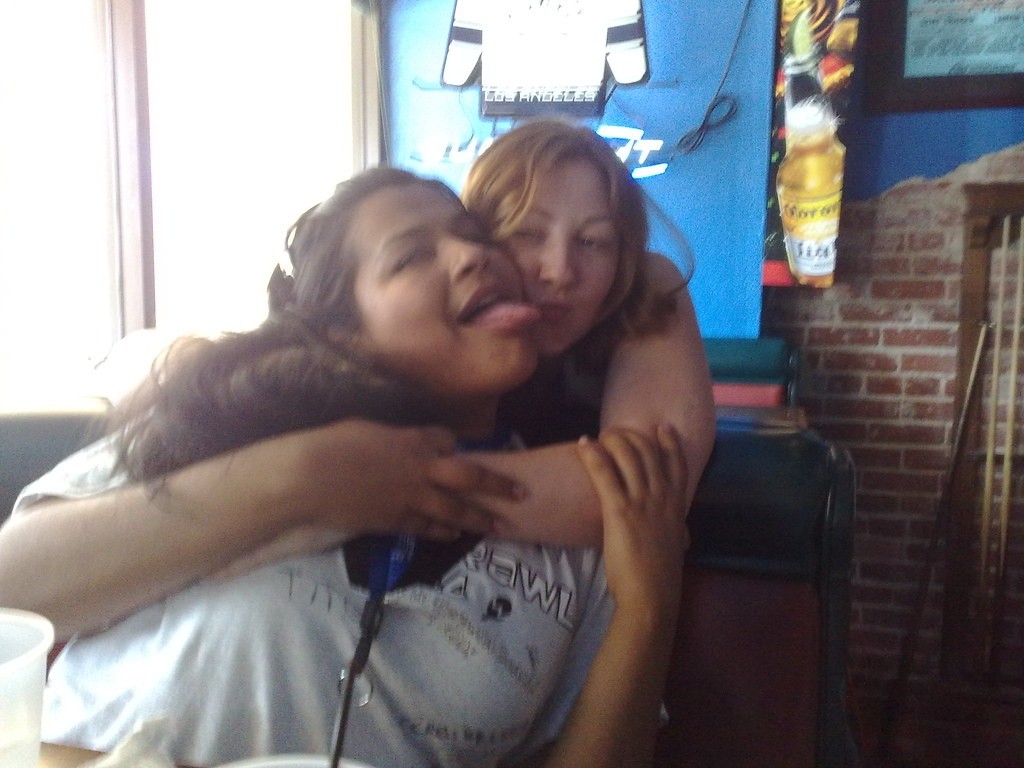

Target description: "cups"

left=0, top=605, right=55, bottom=768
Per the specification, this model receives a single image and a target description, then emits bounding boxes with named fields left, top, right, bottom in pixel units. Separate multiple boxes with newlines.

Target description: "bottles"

left=774, top=52, right=847, bottom=287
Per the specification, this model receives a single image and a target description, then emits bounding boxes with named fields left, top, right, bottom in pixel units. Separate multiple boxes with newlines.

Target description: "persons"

left=2, top=121, right=719, bottom=767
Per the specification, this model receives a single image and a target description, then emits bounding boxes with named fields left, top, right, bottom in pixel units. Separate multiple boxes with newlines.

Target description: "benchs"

left=1, top=337, right=872, bottom=768
